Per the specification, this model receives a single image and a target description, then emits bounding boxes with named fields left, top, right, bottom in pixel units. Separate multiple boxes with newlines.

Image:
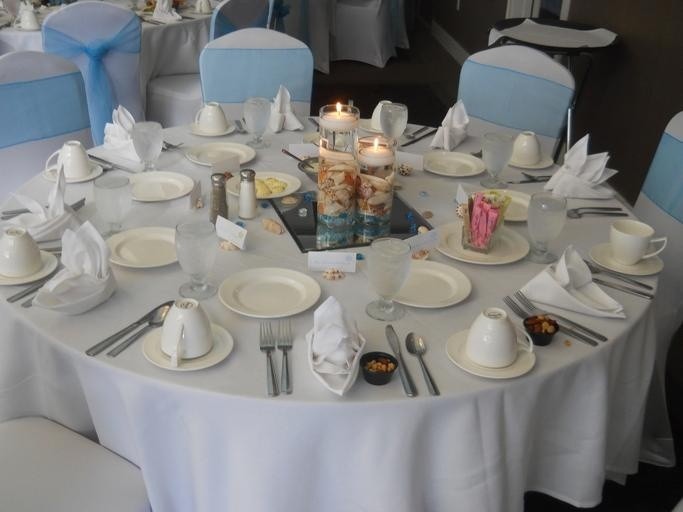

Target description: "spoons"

left=107, top=305, right=170, bottom=358
left=281, top=149, right=316, bottom=169
left=568, top=212, right=628, bottom=219
left=404, top=331, right=440, bottom=397
left=567, top=207, right=621, bottom=215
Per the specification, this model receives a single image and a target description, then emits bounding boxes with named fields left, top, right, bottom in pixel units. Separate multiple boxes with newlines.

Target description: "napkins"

left=431, top=97, right=471, bottom=154
left=8, top=165, right=89, bottom=243
left=270, top=81, right=306, bottom=135
left=100, top=100, right=151, bottom=168
left=545, top=131, right=620, bottom=208
left=31, top=220, right=121, bottom=322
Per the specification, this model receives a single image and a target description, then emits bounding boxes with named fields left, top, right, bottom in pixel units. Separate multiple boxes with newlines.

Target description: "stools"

left=0, top=414, right=148, bottom=512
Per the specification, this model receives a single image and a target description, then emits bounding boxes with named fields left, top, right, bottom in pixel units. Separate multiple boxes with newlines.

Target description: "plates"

left=423, top=151, right=485, bottom=177
left=2, top=249, right=58, bottom=286
left=218, top=266, right=322, bottom=318
left=455, top=189, right=531, bottom=223
left=104, top=227, right=178, bottom=269
left=128, top=171, right=194, bottom=201
left=185, top=142, right=256, bottom=166
left=446, top=329, right=537, bottom=380
left=437, top=224, right=530, bottom=265
left=142, top=323, right=233, bottom=371
left=225, top=172, right=302, bottom=199
left=42, top=162, right=104, bottom=183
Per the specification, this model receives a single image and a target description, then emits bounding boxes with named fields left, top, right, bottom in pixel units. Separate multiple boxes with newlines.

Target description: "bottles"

left=238, top=169, right=256, bottom=219
left=208, top=172, right=227, bottom=222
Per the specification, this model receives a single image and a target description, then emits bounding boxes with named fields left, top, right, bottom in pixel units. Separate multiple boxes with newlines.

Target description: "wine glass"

left=391, top=259, right=473, bottom=307
left=480, top=132, right=508, bottom=188
left=132, top=122, right=164, bottom=171
left=243, top=97, right=272, bottom=148
left=367, top=236, right=411, bottom=321
left=92, top=175, right=133, bottom=239
left=379, top=103, right=407, bottom=150
left=174, top=219, right=218, bottom=300
left=527, top=192, right=567, bottom=265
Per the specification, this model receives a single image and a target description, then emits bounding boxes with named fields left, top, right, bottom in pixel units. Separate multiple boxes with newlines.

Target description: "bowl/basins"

left=299, top=157, right=317, bottom=182
left=2, top=227, right=42, bottom=278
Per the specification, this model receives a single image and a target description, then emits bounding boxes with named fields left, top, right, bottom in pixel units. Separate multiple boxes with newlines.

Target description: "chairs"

left=198, top=24, right=315, bottom=135
left=634, top=112, right=683, bottom=374
left=328, top=1, right=411, bottom=68
left=0, top=48, right=92, bottom=206
left=148, top=1, right=292, bottom=129
left=455, top=44, right=577, bottom=176
left=40, top=0, right=151, bottom=150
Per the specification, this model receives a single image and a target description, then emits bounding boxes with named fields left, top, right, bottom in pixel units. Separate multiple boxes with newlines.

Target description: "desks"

left=485, top=18, right=621, bottom=116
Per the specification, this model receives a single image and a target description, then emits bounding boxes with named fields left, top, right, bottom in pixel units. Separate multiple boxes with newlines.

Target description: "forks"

left=163, top=141, right=184, bottom=149
left=590, top=244, right=662, bottom=275
left=403, top=126, right=428, bottom=139
left=519, top=172, right=553, bottom=179
left=513, top=290, right=608, bottom=342
left=258, top=319, right=281, bottom=399
left=502, top=295, right=600, bottom=347
left=273, top=317, right=294, bottom=394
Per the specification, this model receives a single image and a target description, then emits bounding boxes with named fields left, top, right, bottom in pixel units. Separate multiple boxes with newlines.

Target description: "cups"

left=161, top=298, right=213, bottom=369
left=46, top=140, right=91, bottom=179
left=371, top=100, right=392, bottom=131
left=609, top=219, right=667, bottom=266
left=195, top=0, right=211, bottom=14
left=13, top=10, right=40, bottom=31
left=507, top=153, right=554, bottom=169
left=193, top=101, right=227, bottom=133
left=513, top=130, right=541, bottom=164
left=467, top=308, right=533, bottom=368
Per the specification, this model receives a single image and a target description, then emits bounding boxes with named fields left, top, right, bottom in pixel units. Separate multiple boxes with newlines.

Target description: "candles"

left=358, top=135, right=397, bottom=236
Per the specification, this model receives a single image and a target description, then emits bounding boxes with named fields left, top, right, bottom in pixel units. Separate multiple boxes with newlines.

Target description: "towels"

left=521, top=245, right=626, bottom=323
left=36, top=223, right=116, bottom=312
left=306, top=295, right=363, bottom=395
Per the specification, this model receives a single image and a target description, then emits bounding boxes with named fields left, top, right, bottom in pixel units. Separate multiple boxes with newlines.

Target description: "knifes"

left=384, top=324, right=419, bottom=396
left=583, top=259, right=655, bottom=289
left=400, top=128, right=437, bottom=147
left=9, top=267, right=62, bottom=303
left=592, top=278, right=654, bottom=300
left=86, top=300, right=175, bottom=357
